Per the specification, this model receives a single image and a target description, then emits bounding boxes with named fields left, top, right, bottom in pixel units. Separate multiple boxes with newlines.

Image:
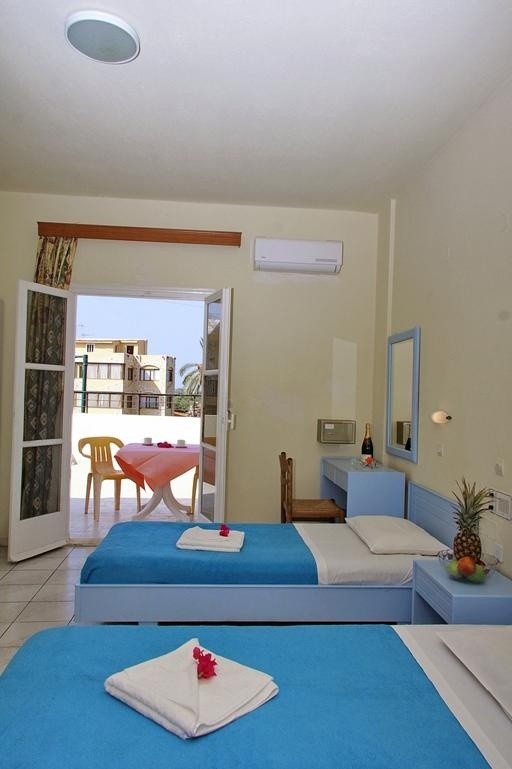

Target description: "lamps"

left=65, top=10, right=141, bottom=64
left=430, top=411, right=453, bottom=425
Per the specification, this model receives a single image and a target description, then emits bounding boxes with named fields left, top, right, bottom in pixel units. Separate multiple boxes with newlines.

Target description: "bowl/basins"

left=436, top=548, right=500, bottom=585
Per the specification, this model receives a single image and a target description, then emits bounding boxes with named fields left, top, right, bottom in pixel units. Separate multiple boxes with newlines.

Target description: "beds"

left=74, top=482, right=467, bottom=626
left=2, top=626, right=511, bottom=769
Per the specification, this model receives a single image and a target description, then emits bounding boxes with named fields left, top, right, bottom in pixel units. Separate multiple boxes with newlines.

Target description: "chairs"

left=278, top=452, right=347, bottom=523
left=77, top=437, right=142, bottom=522
left=190, top=465, right=200, bottom=514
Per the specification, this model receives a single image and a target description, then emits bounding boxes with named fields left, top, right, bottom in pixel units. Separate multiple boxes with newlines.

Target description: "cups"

left=144, top=438, right=152, bottom=444
left=176, top=440, right=186, bottom=447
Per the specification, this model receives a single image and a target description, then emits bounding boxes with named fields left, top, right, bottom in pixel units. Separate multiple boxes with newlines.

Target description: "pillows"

left=347, top=516, right=451, bottom=556
left=435, top=627, right=512, bottom=723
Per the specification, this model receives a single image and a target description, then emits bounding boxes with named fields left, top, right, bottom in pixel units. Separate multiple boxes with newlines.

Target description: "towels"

left=176, top=526, right=246, bottom=553
left=104, top=638, right=278, bottom=738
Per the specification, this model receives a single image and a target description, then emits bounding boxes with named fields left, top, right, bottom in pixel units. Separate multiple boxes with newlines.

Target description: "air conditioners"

left=252, top=237, right=345, bottom=273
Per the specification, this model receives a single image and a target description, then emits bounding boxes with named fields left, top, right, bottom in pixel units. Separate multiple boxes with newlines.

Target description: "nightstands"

left=411, top=559, right=511, bottom=626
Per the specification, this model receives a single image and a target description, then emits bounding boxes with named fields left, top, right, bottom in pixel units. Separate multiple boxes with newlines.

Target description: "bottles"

left=404, top=424, right=411, bottom=451
left=360, top=423, right=374, bottom=466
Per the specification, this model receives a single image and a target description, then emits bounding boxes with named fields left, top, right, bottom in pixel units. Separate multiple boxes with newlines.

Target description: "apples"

left=449, top=557, right=488, bottom=582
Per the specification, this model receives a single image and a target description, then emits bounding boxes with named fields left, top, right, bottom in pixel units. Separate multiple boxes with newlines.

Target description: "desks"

left=115, top=442, right=199, bottom=522
left=318, top=454, right=406, bottom=520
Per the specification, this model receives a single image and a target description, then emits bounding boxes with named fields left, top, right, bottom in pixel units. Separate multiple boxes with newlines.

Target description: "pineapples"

left=452, top=478, right=495, bottom=565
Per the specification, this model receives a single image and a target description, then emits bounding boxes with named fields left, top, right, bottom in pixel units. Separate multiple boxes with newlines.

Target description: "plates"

left=143, top=444, right=153, bottom=446
left=176, top=446, right=187, bottom=448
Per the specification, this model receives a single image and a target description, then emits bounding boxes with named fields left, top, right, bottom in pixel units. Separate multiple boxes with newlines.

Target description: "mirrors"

left=385, top=327, right=420, bottom=465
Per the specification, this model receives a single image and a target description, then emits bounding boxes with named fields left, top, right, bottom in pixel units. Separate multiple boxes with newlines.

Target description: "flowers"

left=219, top=524, right=230, bottom=537
left=191, top=647, right=219, bottom=680
